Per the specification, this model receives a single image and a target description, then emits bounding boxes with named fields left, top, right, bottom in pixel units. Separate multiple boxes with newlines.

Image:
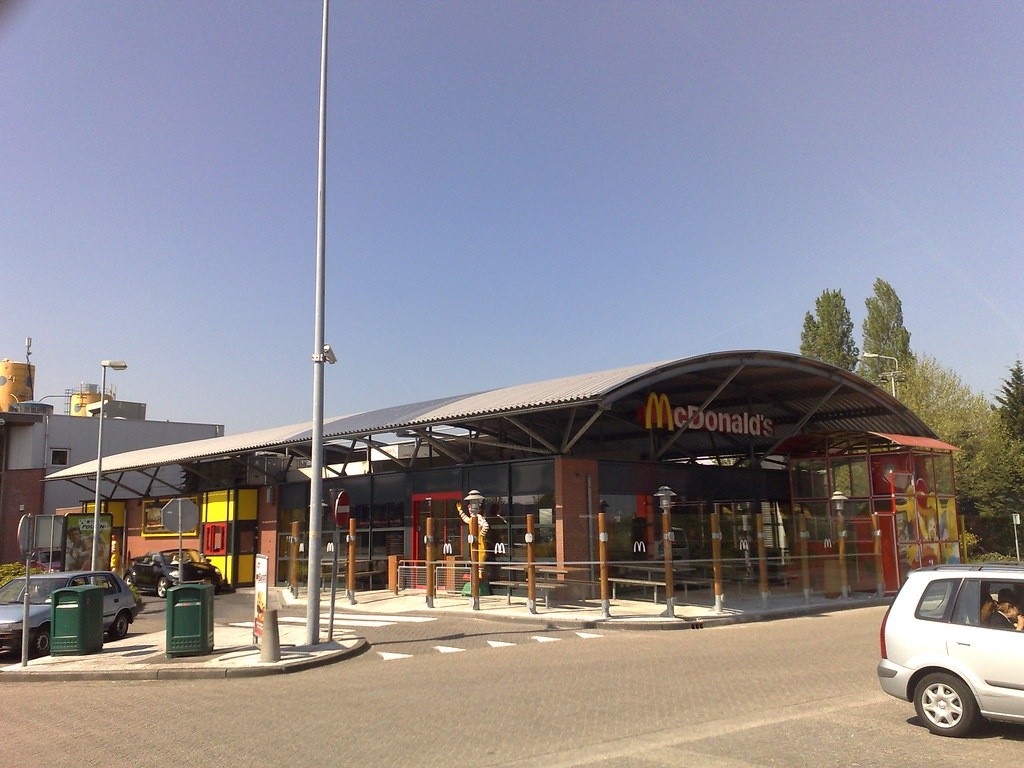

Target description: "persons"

left=981, top=594, right=1024, bottom=632
left=64, top=527, right=109, bottom=571
left=456, top=502, right=489, bottom=582
left=109, top=535, right=121, bottom=572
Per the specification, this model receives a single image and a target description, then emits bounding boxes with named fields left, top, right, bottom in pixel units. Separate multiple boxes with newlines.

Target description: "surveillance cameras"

left=324, top=344, right=338, bottom=364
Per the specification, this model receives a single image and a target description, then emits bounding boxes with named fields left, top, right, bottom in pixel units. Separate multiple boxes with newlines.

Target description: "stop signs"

left=335, top=491, right=350, bottom=528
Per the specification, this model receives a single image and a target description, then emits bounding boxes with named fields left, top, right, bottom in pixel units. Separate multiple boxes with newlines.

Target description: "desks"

left=501, top=565, right=592, bottom=608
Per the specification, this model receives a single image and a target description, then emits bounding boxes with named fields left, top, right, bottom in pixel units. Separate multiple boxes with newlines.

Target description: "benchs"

left=524, top=578, right=601, bottom=605
left=489, top=580, right=568, bottom=609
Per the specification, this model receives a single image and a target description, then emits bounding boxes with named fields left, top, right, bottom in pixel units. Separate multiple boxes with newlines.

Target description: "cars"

left=0, top=570, right=136, bottom=654
left=122, top=549, right=222, bottom=598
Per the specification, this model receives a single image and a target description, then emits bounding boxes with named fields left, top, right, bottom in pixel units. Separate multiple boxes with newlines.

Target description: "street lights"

left=91, top=360, right=128, bottom=584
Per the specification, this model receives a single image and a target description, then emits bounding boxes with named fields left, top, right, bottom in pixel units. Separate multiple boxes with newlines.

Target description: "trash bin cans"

left=49, top=585, right=105, bottom=658
left=165, top=584, right=216, bottom=659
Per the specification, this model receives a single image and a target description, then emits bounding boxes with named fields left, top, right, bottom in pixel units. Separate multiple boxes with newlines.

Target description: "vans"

left=654, top=528, right=691, bottom=572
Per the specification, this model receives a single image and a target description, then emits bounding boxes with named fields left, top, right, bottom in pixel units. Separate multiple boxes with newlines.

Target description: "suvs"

left=877, top=562, right=1024, bottom=736
